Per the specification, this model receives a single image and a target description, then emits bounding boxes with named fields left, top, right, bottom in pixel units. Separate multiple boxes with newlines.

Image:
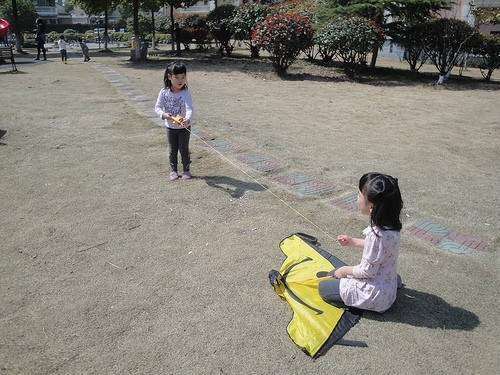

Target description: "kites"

left=268, top=232, right=369, bottom=359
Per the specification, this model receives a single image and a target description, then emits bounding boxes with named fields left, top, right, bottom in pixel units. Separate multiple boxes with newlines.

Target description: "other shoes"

left=169, top=171, right=178, bottom=180
left=182, top=171, right=191, bottom=179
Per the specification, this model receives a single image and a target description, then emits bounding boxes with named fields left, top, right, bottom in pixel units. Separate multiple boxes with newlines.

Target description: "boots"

left=35, top=53, right=40, bottom=59
left=42, top=54, right=47, bottom=61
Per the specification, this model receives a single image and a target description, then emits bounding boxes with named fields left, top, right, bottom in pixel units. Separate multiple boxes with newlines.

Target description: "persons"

left=77, top=38, right=91, bottom=62
left=58, top=35, right=68, bottom=64
left=33, top=18, right=47, bottom=61
left=318, top=172, right=404, bottom=313
left=155, top=61, right=194, bottom=181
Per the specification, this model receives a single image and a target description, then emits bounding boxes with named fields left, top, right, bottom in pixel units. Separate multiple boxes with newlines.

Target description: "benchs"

left=0, top=45, right=17, bottom=72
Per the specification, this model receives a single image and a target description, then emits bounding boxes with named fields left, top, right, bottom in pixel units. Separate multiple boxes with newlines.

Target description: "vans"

left=94, top=28, right=105, bottom=33
